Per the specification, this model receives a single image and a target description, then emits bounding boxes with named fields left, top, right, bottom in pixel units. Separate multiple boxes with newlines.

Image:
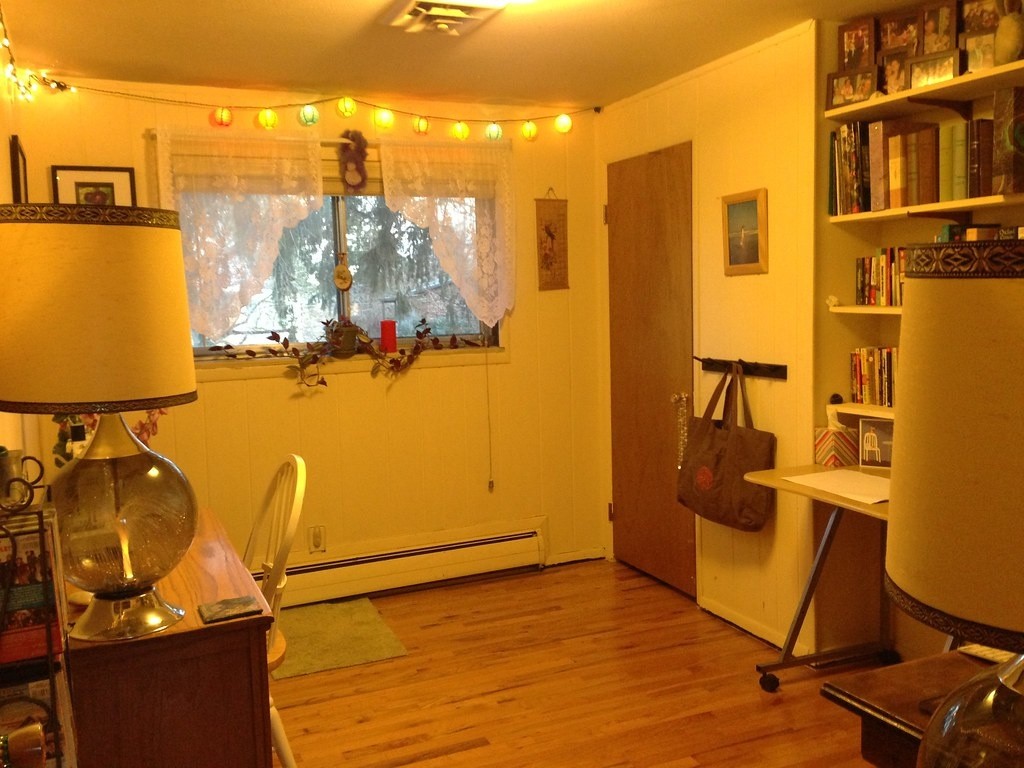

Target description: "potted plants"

left=208, top=315, right=477, bottom=386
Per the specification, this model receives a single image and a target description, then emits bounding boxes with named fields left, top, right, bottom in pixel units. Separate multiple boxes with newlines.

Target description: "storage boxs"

left=869, top=120, right=938, bottom=211
left=991, top=86, right=1024, bottom=195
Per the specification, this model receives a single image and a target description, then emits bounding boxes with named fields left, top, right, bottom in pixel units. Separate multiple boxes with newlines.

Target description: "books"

left=850, top=346, right=897, bottom=407
left=828, top=86, right=1024, bottom=216
left=856, top=220, right=1024, bottom=305
left=0, top=508, right=77, bottom=768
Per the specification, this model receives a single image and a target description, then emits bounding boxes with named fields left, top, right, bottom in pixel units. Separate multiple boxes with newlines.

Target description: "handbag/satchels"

left=677, top=362, right=777, bottom=532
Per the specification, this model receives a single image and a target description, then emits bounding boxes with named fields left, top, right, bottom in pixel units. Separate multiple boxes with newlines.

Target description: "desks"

left=66, top=507, right=276, bottom=768
left=820, top=646, right=1024, bottom=768
left=743, top=462, right=890, bottom=690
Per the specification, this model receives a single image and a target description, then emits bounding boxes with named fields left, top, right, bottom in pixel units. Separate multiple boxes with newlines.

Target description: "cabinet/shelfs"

left=0, top=504, right=79, bottom=768
left=822, top=0, right=1024, bottom=662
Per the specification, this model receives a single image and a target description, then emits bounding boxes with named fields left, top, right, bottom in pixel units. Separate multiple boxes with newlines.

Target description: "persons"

left=824, top=2, right=1006, bottom=104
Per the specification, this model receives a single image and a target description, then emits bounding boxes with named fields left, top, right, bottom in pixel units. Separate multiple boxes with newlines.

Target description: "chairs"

left=242, top=454, right=307, bottom=768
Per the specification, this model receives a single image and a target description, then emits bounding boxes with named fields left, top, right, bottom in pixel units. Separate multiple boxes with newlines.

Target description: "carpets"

left=271, top=596, right=410, bottom=680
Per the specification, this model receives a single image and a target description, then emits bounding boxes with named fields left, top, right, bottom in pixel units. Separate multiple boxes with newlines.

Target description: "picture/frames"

left=877, top=42, right=914, bottom=94
left=825, top=64, right=879, bottom=111
left=720, top=187, right=770, bottom=276
left=879, top=9, right=920, bottom=58
left=918, top=0, right=959, bottom=57
left=10, top=135, right=28, bottom=204
left=904, top=47, right=961, bottom=90
left=51, top=164, right=138, bottom=208
left=959, top=24, right=998, bottom=75
left=837, top=16, right=877, bottom=73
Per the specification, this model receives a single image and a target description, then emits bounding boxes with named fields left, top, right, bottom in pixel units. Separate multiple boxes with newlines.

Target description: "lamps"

left=451, top=120, right=471, bottom=142
left=485, top=121, right=503, bottom=141
left=0, top=204, right=198, bottom=642
left=214, top=107, right=234, bottom=126
left=298, top=104, right=319, bottom=124
left=520, top=121, right=538, bottom=140
left=879, top=239, right=1024, bottom=724
left=372, top=108, right=395, bottom=129
left=413, top=116, right=433, bottom=137
left=555, top=114, right=572, bottom=133
left=337, top=97, right=357, bottom=118
left=258, top=108, right=279, bottom=130
